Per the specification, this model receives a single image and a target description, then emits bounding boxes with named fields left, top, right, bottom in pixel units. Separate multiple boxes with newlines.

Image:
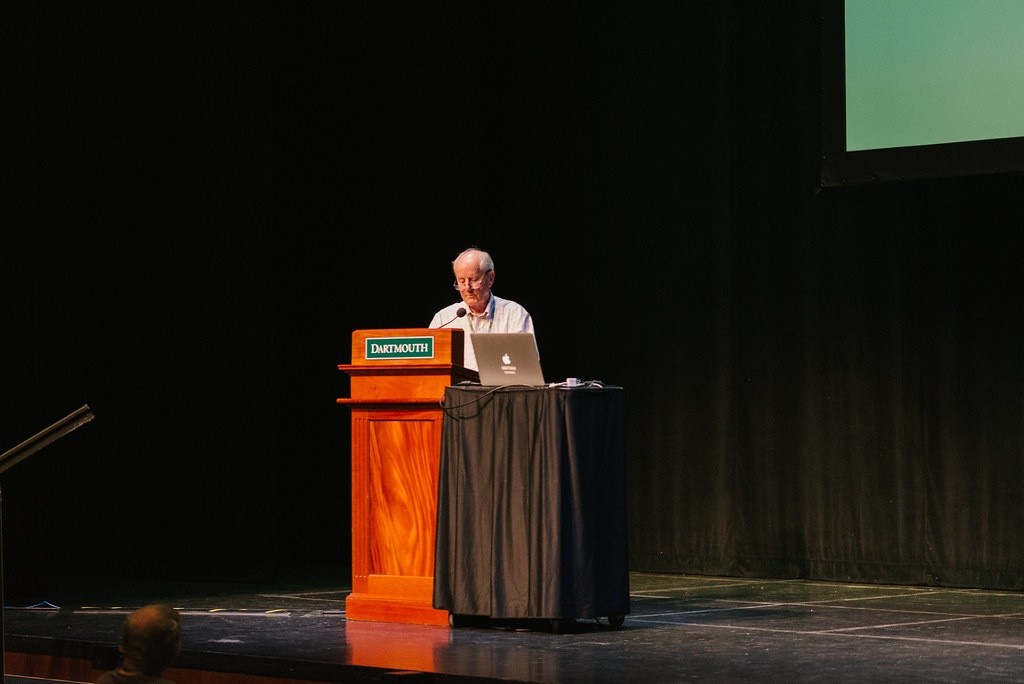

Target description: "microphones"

left=439, top=307, right=466, bottom=328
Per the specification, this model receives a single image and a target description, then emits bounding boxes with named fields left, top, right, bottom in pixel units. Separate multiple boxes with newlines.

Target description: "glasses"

left=453, top=269, right=492, bottom=291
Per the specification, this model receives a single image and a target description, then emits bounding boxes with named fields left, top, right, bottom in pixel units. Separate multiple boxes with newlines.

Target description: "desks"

left=431, top=385, right=633, bottom=631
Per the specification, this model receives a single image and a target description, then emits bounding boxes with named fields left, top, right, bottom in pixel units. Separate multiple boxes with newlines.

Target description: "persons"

left=428, top=248, right=540, bottom=372
left=96, top=603, right=183, bottom=684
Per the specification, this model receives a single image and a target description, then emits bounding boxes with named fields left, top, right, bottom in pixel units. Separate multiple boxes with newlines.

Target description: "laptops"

left=470, top=332, right=550, bottom=387
